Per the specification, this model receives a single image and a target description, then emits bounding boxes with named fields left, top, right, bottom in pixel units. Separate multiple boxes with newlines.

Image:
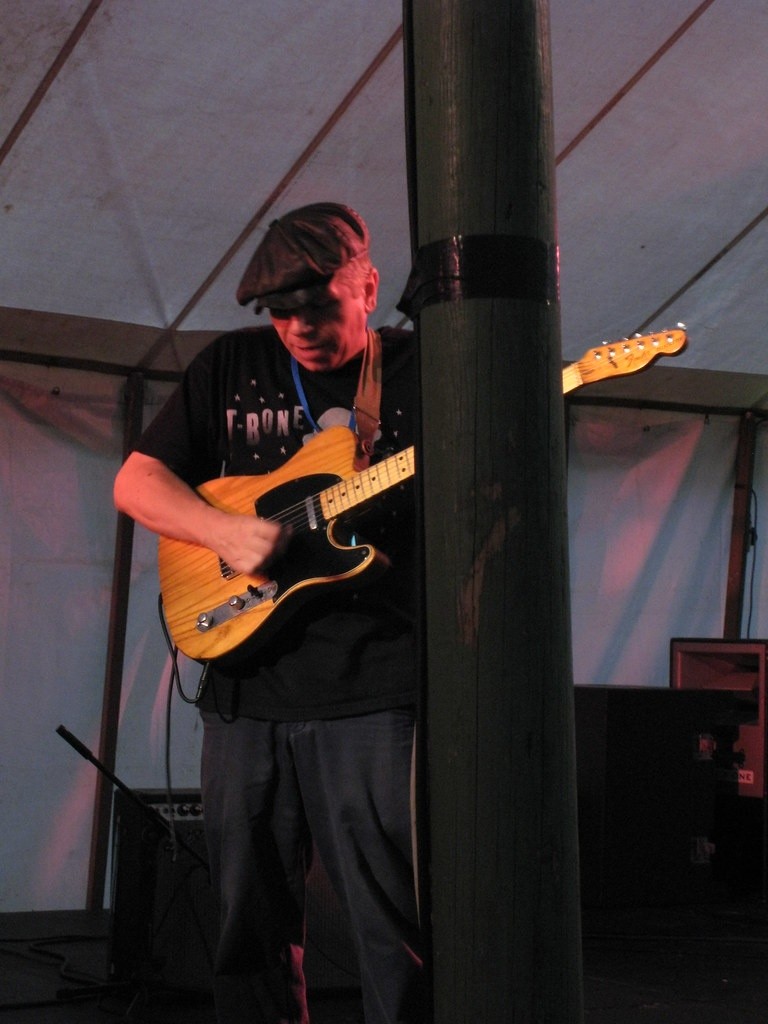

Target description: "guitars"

left=157, top=322, right=687, bottom=679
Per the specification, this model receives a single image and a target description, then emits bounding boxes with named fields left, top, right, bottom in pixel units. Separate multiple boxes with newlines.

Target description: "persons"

left=112, top=202, right=421, bottom=1024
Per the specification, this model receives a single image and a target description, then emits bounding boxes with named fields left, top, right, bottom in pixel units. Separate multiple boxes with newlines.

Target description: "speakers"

left=575, top=684, right=719, bottom=912
left=671, top=638, right=768, bottom=798
left=114, top=787, right=363, bottom=995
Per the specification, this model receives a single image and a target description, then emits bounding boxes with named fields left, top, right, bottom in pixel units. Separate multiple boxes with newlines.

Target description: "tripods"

left=53, top=726, right=216, bottom=1024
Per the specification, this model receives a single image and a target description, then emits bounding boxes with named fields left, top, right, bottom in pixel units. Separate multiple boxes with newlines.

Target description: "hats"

left=236, top=199, right=370, bottom=312
